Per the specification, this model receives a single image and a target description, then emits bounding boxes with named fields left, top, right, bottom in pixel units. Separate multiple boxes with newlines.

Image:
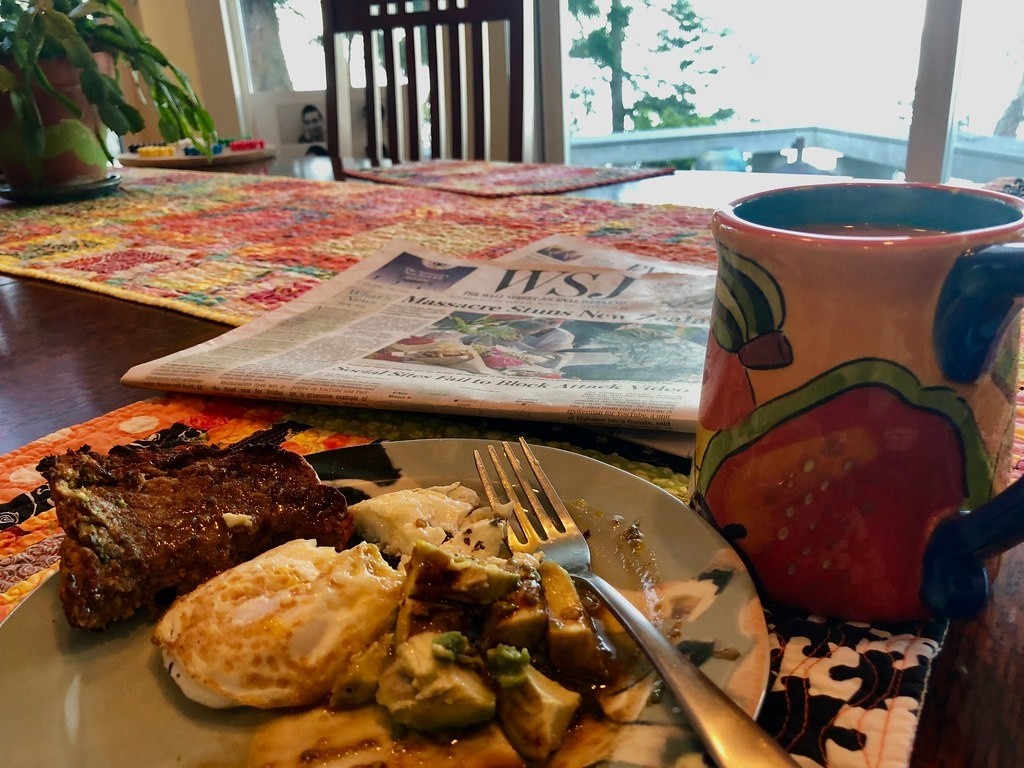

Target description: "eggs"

left=152, top=537, right=408, bottom=710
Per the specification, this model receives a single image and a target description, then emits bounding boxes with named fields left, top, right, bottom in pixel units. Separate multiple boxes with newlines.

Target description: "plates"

left=0, top=438, right=772, bottom=768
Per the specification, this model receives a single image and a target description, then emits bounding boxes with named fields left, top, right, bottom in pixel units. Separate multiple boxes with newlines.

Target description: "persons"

left=363, top=101, right=391, bottom=158
left=307, top=145, right=326, bottom=156
left=296, top=104, right=329, bottom=144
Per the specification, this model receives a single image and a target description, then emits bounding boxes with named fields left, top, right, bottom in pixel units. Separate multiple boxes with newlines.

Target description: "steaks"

left=35, top=443, right=356, bottom=631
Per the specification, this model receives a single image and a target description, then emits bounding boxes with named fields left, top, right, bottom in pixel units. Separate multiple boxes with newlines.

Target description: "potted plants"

left=0, top=0, right=219, bottom=203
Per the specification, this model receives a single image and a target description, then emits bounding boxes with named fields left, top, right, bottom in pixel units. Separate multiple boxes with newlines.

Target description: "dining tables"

left=0, top=165, right=1024, bottom=768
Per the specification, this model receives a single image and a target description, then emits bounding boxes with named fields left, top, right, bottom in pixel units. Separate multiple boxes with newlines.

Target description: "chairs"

left=319, top=0, right=535, bottom=165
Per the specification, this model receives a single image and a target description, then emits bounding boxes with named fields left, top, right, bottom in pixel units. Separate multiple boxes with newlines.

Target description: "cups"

left=685, top=182, right=1024, bottom=627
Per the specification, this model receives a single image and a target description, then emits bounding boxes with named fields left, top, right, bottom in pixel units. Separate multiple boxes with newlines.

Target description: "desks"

left=118, top=144, right=277, bottom=175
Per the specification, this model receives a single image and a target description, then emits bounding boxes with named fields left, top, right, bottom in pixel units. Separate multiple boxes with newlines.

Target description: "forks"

left=474, top=435, right=803, bottom=767
